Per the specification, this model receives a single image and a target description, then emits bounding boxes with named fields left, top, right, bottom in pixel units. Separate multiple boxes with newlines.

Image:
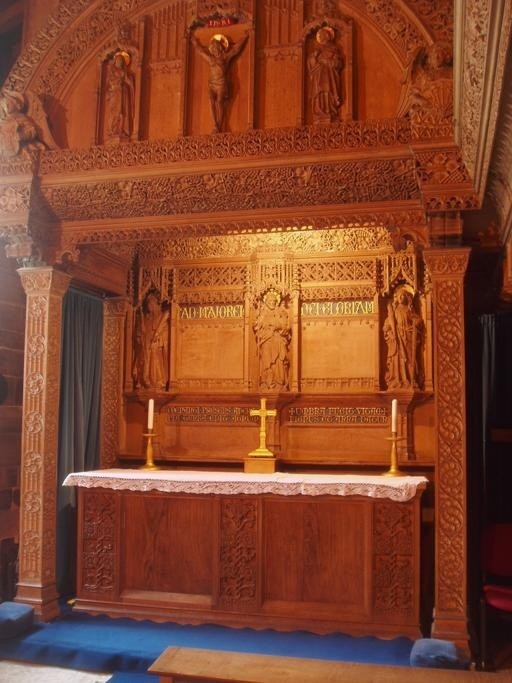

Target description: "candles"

left=147, top=398, right=155, bottom=430
left=390, top=398, right=397, bottom=433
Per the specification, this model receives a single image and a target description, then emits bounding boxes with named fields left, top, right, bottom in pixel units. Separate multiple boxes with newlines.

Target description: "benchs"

left=145, top=645, right=511, bottom=682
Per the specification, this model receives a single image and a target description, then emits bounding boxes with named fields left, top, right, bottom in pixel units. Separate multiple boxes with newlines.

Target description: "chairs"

left=471, top=520, right=512, bottom=669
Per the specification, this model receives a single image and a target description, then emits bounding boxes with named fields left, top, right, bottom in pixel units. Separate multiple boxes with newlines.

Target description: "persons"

left=379, top=288, right=426, bottom=390
left=249, top=292, right=293, bottom=391
left=307, top=25, right=346, bottom=118
left=0, top=87, right=48, bottom=153
left=397, top=39, right=454, bottom=116
left=103, top=54, right=135, bottom=136
left=186, top=28, right=251, bottom=133
left=130, top=292, right=170, bottom=391
